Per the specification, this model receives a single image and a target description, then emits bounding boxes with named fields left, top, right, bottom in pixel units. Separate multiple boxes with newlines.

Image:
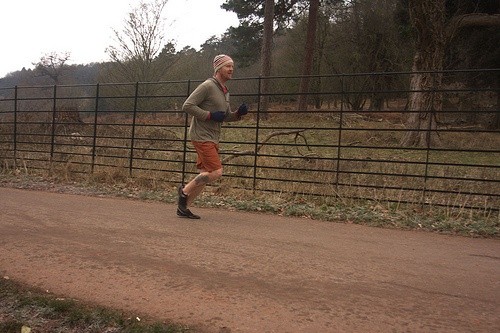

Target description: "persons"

left=176, top=54, right=248, bottom=219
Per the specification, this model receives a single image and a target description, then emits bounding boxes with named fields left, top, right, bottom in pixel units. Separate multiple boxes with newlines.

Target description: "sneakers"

left=176, top=187, right=201, bottom=220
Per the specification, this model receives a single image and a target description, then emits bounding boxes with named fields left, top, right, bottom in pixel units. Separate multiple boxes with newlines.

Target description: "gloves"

left=238, top=103, right=249, bottom=115
left=210, top=111, right=226, bottom=122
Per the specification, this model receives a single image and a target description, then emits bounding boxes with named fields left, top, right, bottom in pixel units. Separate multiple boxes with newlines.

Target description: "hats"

left=214, top=54, right=234, bottom=72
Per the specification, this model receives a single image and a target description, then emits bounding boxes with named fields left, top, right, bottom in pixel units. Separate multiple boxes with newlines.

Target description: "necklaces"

left=212, top=76, right=230, bottom=102
